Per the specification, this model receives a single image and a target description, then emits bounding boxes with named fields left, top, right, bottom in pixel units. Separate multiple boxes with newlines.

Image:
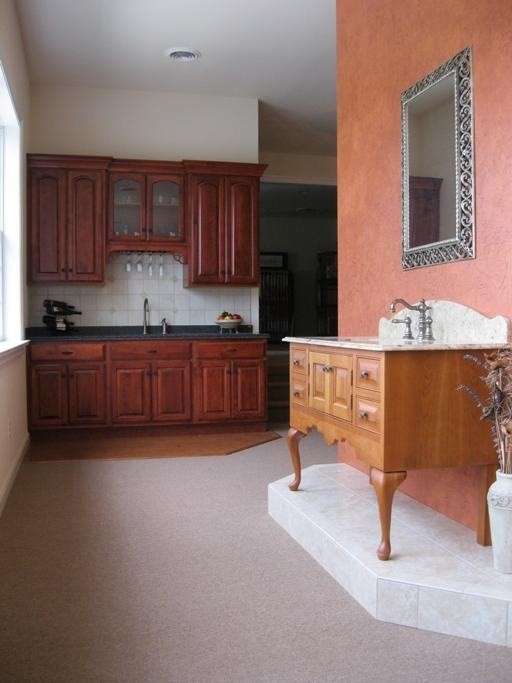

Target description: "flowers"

left=456, top=343, right=512, bottom=474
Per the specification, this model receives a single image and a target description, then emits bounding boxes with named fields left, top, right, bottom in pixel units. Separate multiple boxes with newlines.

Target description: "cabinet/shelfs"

left=409, top=176, right=444, bottom=248
left=286, top=342, right=512, bottom=560
left=193, top=339, right=269, bottom=435
left=28, top=341, right=110, bottom=441
left=26, top=153, right=113, bottom=285
left=106, top=159, right=190, bottom=264
left=110, top=340, right=193, bottom=437
left=183, top=160, right=268, bottom=288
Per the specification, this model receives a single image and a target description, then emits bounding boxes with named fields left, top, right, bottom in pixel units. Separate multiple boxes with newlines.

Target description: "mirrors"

left=400, top=47, right=476, bottom=270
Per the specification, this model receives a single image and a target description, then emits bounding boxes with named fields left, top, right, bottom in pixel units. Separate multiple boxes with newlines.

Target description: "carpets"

left=26, top=431, right=281, bottom=461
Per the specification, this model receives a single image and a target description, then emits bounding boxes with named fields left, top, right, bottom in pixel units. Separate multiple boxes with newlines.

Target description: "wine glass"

left=122, top=252, right=165, bottom=279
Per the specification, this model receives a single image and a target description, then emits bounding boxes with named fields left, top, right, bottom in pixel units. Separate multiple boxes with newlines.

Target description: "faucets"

left=388, top=298, right=431, bottom=341
left=143, top=298, right=149, bottom=335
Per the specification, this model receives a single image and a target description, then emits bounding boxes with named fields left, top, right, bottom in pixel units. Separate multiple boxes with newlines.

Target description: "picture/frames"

left=259, top=251, right=288, bottom=269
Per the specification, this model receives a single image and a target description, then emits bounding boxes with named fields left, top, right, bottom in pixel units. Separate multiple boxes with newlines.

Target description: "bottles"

left=42, top=299, right=82, bottom=333
